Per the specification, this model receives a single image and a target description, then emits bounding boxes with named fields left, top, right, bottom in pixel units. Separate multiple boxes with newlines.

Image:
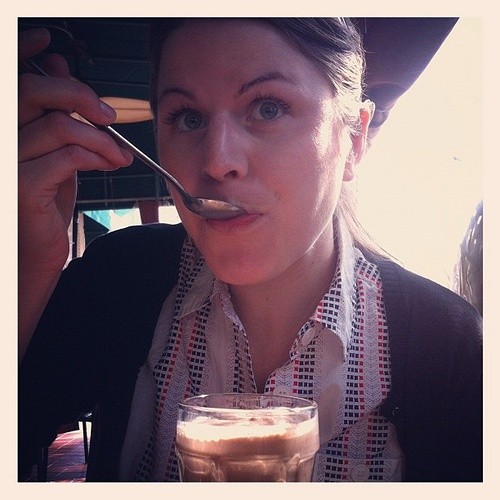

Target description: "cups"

left=174, top=393, right=319, bottom=483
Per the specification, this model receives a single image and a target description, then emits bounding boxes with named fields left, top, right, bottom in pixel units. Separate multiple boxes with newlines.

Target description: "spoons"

left=24, top=57, right=247, bottom=220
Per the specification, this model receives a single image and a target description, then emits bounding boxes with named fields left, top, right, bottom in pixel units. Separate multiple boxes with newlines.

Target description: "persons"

left=18, top=16, right=481, bottom=482
